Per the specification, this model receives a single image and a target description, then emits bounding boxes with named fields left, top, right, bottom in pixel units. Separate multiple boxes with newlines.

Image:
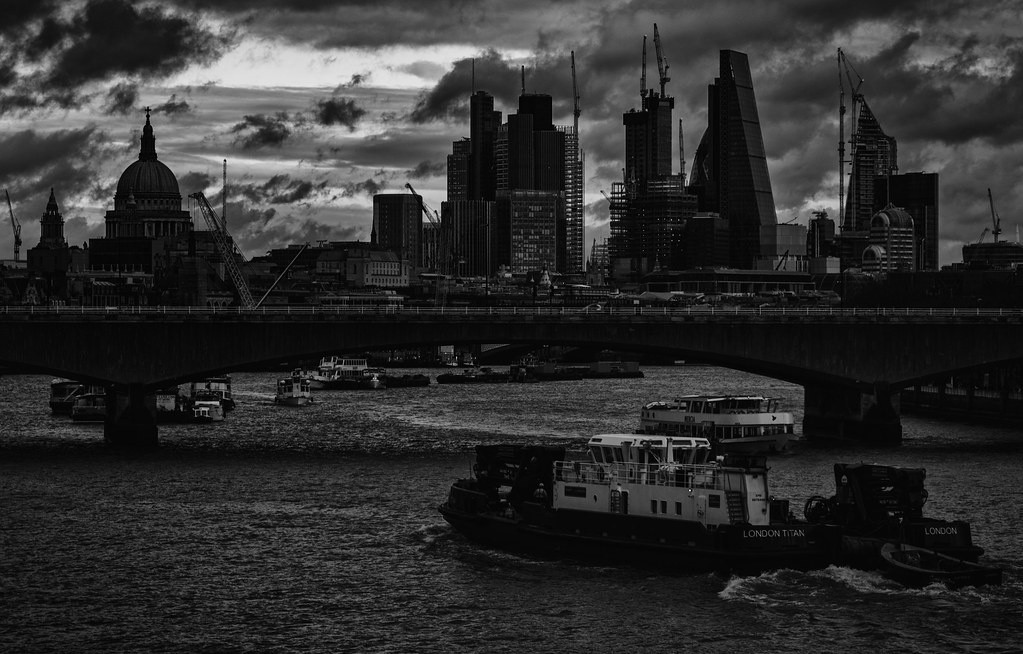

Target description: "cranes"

left=405, top=183, right=441, bottom=236
left=836, top=47, right=866, bottom=230
left=187, top=191, right=276, bottom=311
left=5, top=189, right=22, bottom=260
left=988, top=187, right=1002, bottom=243
left=639, top=22, right=672, bottom=111
left=570, top=49, right=582, bottom=133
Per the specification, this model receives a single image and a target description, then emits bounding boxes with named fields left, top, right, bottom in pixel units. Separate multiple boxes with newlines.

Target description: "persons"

left=533, top=483, right=547, bottom=508
left=836, top=475, right=854, bottom=519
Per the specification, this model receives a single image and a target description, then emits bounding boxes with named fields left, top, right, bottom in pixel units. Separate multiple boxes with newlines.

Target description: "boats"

left=435, top=430, right=850, bottom=582
left=155, top=372, right=237, bottom=424
left=436, top=352, right=578, bottom=385
left=48, top=377, right=106, bottom=424
left=274, top=367, right=315, bottom=407
left=633, top=393, right=803, bottom=468
left=879, top=541, right=1004, bottom=591
left=307, top=346, right=431, bottom=390
